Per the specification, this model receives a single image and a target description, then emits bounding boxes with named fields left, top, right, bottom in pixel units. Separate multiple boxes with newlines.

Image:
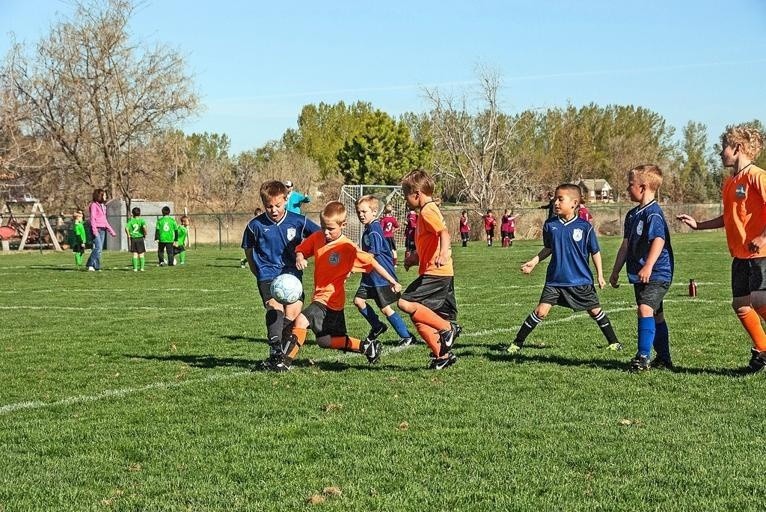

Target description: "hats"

left=285, top=181, right=293, bottom=187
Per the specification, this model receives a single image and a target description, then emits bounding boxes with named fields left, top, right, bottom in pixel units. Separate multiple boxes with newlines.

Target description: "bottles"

left=688, top=278, right=697, bottom=297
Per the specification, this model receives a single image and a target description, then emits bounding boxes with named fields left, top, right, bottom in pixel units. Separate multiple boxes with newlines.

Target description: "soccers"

left=270, top=274, right=304, bottom=304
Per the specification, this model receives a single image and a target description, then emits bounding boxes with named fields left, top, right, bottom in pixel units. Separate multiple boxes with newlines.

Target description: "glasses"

left=287, top=186, right=289, bottom=188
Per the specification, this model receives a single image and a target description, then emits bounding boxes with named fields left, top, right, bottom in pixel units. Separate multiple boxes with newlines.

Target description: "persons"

left=252, top=201, right=401, bottom=373
left=380, top=204, right=401, bottom=267
left=507, top=184, right=622, bottom=355
left=353, top=196, right=418, bottom=347
left=579, top=198, right=593, bottom=226
left=398, top=169, right=462, bottom=370
left=676, top=128, right=766, bottom=374
left=173, top=215, right=189, bottom=265
left=402, top=202, right=419, bottom=272
left=507, top=215, right=515, bottom=247
left=609, top=165, right=674, bottom=372
left=284, top=180, right=311, bottom=215
left=474, top=209, right=498, bottom=247
left=125, top=207, right=148, bottom=271
left=72, top=211, right=86, bottom=266
left=460, top=211, right=470, bottom=247
left=241, top=181, right=322, bottom=367
left=85, top=189, right=116, bottom=271
left=501, top=208, right=519, bottom=247
left=154, top=207, right=178, bottom=267
left=241, top=208, right=262, bottom=269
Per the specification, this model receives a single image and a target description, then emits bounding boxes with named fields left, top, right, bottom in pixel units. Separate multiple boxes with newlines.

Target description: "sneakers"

left=429, top=350, right=457, bottom=373
left=650, top=358, right=672, bottom=368
left=439, top=323, right=462, bottom=357
left=505, top=342, right=520, bottom=355
left=394, top=265, right=397, bottom=267
left=745, top=348, right=766, bottom=374
left=241, top=259, right=244, bottom=268
left=259, top=357, right=291, bottom=373
left=629, top=355, right=648, bottom=371
left=606, top=344, right=623, bottom=351
left=159, top=262, right=164, bottom=267
left=362, top=338, right=383, bottom=368
left=366, top=323, right=388, bottom=342
left=85, top=266, right=95, bottom=272
left=270, top=342, right=286, bottom=368
left=131, top=268, right=137, bottom=272
left=396, top=334, right=417, bottom=347
